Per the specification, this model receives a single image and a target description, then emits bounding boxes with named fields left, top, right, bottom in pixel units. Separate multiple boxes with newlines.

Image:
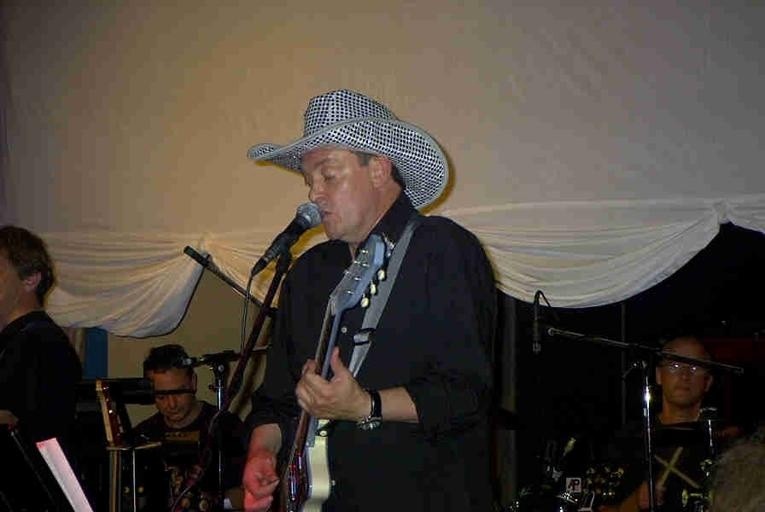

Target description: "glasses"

left=659, top=362, right=706, bottom=376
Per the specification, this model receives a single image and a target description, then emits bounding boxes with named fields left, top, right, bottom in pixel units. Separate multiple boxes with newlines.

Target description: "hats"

left=245, top=88, right=449, bottom=211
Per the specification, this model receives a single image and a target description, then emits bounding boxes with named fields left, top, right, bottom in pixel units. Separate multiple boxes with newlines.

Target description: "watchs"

left=356, top=390, right=382, bottom=434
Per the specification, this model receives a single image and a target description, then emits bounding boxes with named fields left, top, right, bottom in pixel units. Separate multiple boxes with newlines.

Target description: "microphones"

left=176, top=350, right=241, bottom=369
left=250, top=201, right=323, bottom=275
left=531, top=288, right=544, bottom=354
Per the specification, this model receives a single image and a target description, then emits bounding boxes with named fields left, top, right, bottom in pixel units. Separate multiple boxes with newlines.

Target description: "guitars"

left=96, top=378, right=127, bottom=512
left=265, top=233, right=396, bottom=512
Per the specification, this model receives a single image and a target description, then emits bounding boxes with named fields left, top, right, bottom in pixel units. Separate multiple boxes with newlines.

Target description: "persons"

left=591, top=333, right=747, bottom=511
left=124, top=347, right=251, bottom=511
left=0, top=226, right=83, bottom=512
left=258, top=91, right=511, bottom=509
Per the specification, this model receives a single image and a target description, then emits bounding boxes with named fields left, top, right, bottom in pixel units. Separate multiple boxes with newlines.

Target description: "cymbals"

left=667, top=417, right=745, bottom=430
left=101, top=432, right=161, bottom=451
